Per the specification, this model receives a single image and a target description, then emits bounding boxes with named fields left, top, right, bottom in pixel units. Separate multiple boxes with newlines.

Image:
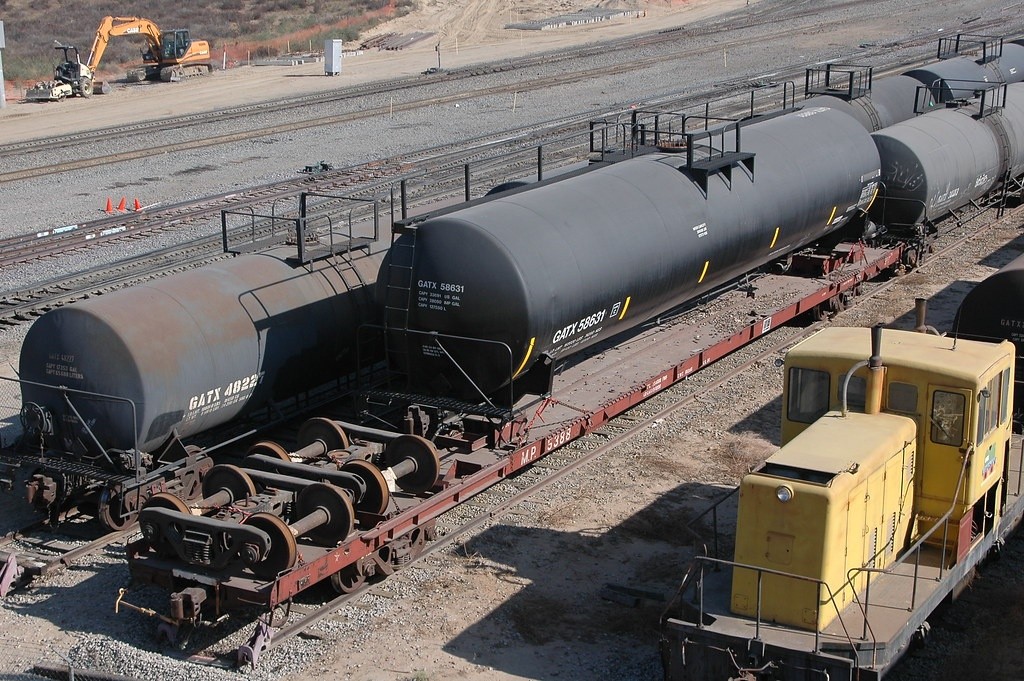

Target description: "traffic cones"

left=106, top=198, right=113, bottom=213
left=116, top=197, right=126, bottom=211
left=134, top=198, right=141, bottom=211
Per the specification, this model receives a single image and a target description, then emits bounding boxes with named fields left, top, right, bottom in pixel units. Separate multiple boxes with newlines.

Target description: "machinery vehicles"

left=25, top=44, right=95, bottom=101
left=85, top=14, right=214, bottom=95
